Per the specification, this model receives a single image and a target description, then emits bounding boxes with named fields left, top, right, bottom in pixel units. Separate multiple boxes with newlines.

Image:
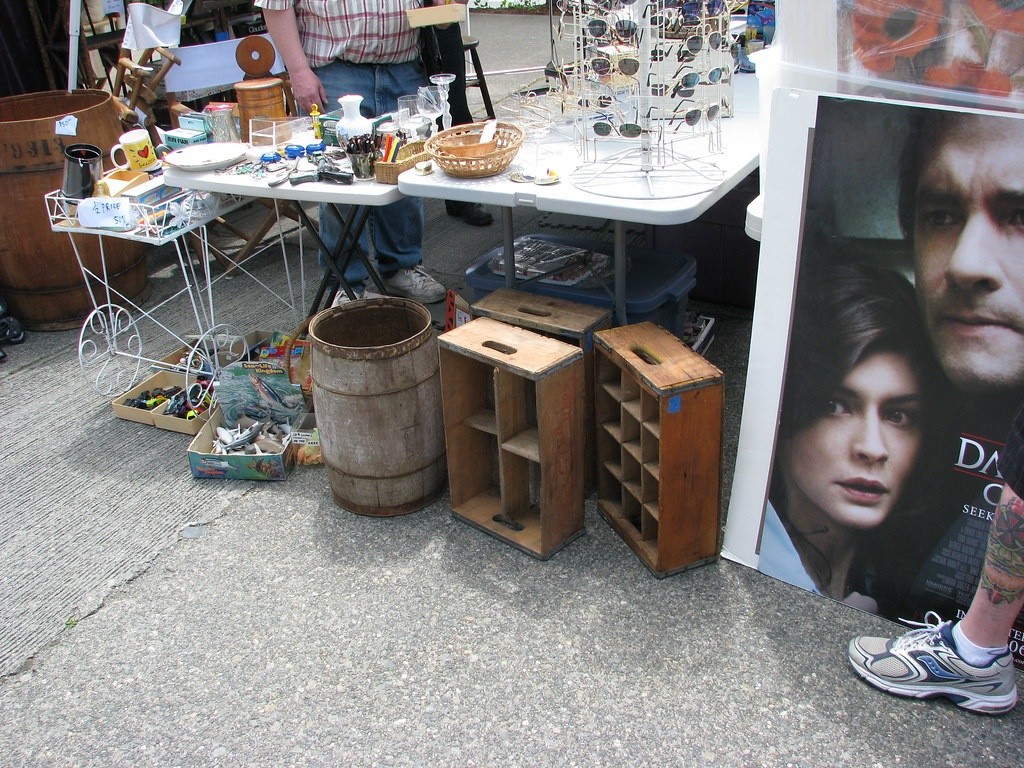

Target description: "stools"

left=461, top=35, right=496, bottom=119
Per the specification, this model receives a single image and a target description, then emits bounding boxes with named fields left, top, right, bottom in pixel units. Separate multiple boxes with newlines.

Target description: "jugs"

left=206, top=109, right=240, bottom=143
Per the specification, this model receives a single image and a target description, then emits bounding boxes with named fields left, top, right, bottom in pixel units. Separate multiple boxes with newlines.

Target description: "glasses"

left=509, top=170, right=559, bottom=184
left=542, top=0, right=734, bottom=144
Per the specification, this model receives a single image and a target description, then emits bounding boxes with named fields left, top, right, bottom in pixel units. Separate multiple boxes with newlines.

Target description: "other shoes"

left=0, top=316, right=26, bottom=344
left=445, top=199, right=493, bottom=227
left=0, top=349, right=7, bottom=361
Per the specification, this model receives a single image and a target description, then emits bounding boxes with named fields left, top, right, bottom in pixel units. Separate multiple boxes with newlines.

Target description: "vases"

left=336, top=94, right=372, bottom=168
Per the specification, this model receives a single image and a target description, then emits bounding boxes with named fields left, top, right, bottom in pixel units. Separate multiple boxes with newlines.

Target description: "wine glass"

left=392, top=73, right=452, bottom=141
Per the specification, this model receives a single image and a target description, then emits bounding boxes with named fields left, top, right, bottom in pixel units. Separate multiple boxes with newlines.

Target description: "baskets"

left=423, top=121, right=526, bottom=179
left=373, top=142, right=432, bottom=185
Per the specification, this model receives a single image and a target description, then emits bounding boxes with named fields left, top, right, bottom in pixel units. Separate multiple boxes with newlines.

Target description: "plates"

left=165, top=144, right=246, bottom=171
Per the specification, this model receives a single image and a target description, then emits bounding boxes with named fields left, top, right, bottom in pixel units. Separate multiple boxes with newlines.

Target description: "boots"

left=731, top=33, right=755, bottom=76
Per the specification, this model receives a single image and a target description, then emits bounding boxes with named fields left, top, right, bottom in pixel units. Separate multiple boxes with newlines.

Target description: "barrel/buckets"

left=309, top=296, right=446, bottom=517
left=0, top=89, right=149, bottom=332
left=59, top=143, right=103, bottom=205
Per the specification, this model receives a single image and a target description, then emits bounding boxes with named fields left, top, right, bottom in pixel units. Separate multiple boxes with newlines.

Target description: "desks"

left=163, top=43, right=781, bottom=339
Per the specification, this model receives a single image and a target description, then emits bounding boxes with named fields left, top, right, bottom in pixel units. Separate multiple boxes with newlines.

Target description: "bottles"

left=335, top=95, right=373, bottom=154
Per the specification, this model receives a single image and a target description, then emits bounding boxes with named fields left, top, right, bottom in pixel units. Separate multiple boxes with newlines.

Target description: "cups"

left=111, top=129, right=158, bottom=172
left=348, top=152, right=377, bottom=180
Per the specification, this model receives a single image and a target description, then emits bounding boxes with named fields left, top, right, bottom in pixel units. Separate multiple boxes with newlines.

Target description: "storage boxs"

left=102, top=116, right=208, bottom=218
left=597, top=44, right=639, bottom=89
left=111, top=331, right=327, bottom=480
left=249, top=118, right=313, bottom=155
left=404, top=3, right=467, bottom=28
left=438, top=168, right=759, bottom=580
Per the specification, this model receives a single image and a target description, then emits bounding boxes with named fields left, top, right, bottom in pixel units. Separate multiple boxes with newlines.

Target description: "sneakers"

left=380, top=264, right=447, bottom=304
left=849, top=610, right=1018, bottom=715
left=330, top=288, right=364, bottom=309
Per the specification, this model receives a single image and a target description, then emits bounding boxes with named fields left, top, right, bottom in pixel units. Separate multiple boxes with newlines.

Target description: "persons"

left=253, top=0, right=456, bottom=308
left=424, top=0, right=493, bottom=226
left=847, top=399, right=1024, bottom=714
left=897, top=107, right=1024, bottom=396
left=755, top=262, right=938, bottom=613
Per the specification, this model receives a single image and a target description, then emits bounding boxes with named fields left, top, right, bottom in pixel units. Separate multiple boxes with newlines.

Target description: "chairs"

left=112, top=0, right=320, bottom=276
